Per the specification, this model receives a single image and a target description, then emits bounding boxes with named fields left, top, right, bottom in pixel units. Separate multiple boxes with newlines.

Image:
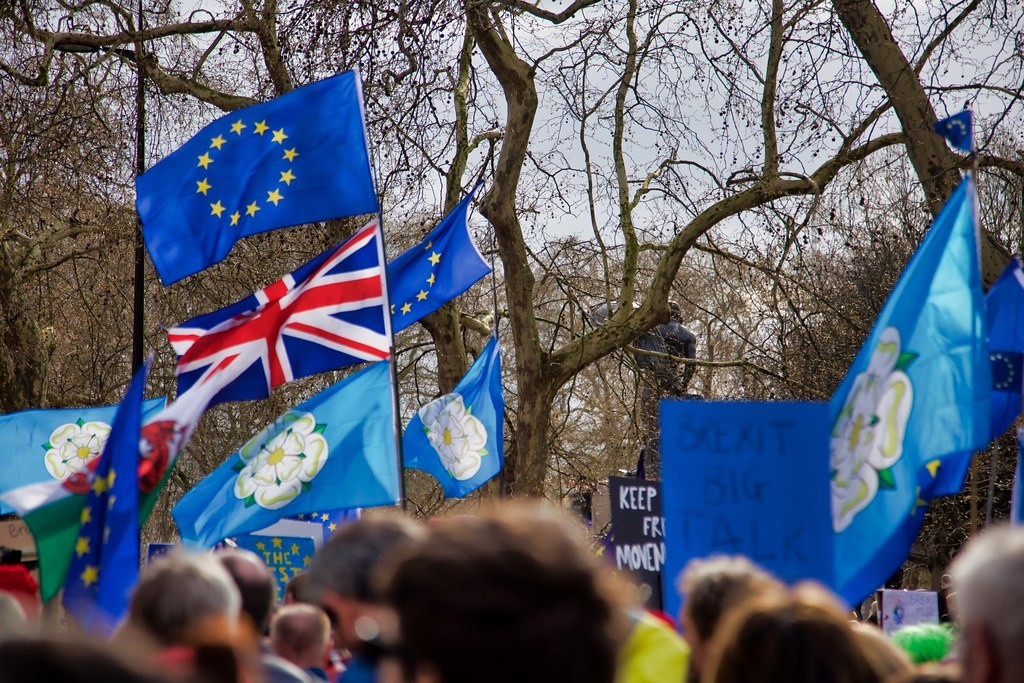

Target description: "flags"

left=0, top=67, right=1024, bottom=636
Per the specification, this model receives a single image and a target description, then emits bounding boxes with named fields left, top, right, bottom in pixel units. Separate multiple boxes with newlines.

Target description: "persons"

left=635, top=300, right=706, bottom=478
left=0, top=494, right=1023, bottom=683
